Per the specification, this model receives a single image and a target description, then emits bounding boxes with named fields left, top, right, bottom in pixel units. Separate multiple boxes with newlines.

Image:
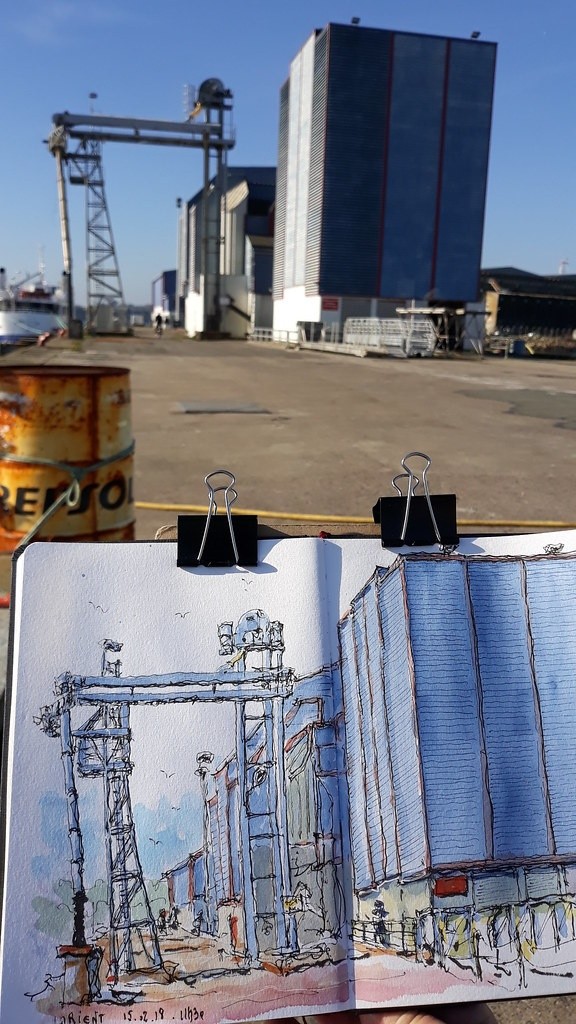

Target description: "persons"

left=156, top=312, right=163, bottom=331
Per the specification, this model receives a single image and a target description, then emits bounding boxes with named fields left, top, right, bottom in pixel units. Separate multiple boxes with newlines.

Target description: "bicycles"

left=156, top=325, right=164, bottom=339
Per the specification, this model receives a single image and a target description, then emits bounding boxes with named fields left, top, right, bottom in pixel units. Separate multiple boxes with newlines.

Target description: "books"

left=0, top=528, right=576, bottom=1024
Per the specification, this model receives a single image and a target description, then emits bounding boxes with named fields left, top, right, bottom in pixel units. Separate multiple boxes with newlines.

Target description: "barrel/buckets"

left=0, top=365, right=135, bottom=555
left=510, top=340, right=524, bottom=355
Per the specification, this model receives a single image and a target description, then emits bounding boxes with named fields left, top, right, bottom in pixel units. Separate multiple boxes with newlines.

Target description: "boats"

left=0, top=267, right=68, bottom=348
